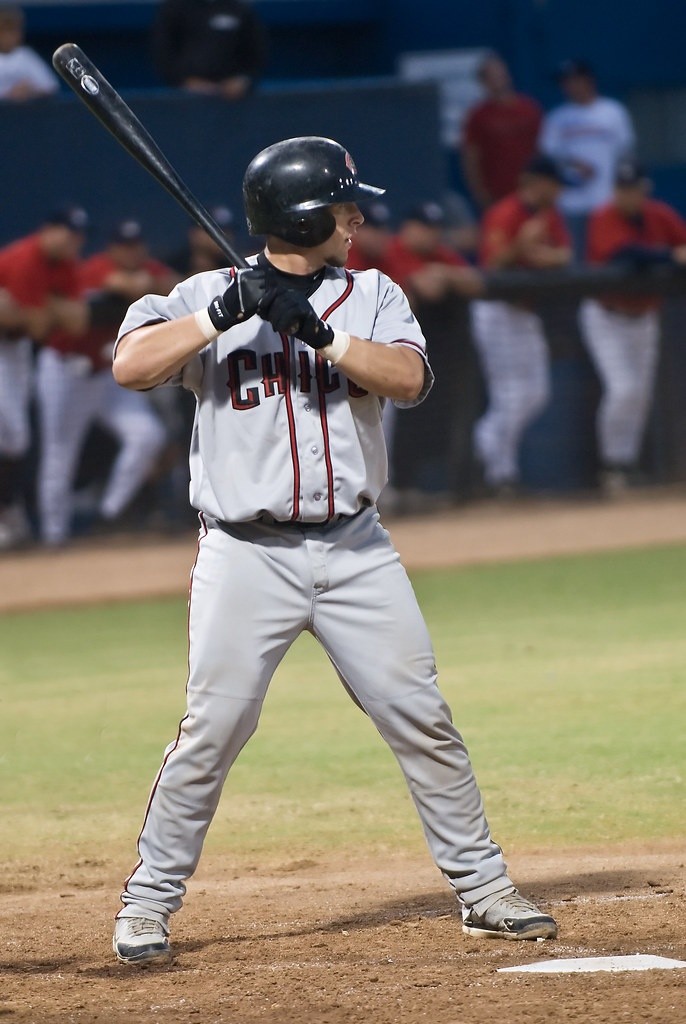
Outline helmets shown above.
[243,137,387,248]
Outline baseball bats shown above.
[51,41,305,339]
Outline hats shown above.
[526,154,576,190]
[205,205,237,230]
[117,218,148,245]
[51,204,91,233]
[614,161,648,186]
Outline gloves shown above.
[208,264,277,332]
[257,290,335,351]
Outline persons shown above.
[0,192,478,558]
[534,62,637,220]
[456,51,541,216]
[112,139,560,964]
[467,153,572,496]
[157,0,258,100]
[577,154,686,491]
[0,0,62,104]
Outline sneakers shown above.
[461,893,556,941]
[114,916,172,965]
